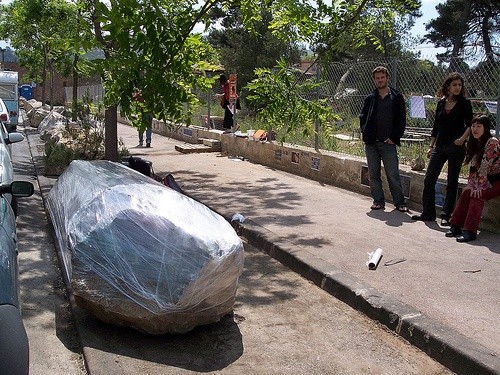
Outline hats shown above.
[219,75,228,84]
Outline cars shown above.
[0,65,34,375]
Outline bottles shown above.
[247,130,255,141]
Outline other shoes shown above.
[441,218,450,226]
[446,227,462,237]
[412,214,435,222]
[233,124,240,133]
[223,129,232,133]
[456,232,476,242]
[146,143,150,147]
[137,144,143,146]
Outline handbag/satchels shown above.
[252,130,277,141]
[220,96,227,109]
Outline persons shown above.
[137,108,153,148]
[214,74,240,134]
[411,73,474,224]
[359,65,408,211]
[445,114,500,242]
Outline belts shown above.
[373,137,386,142]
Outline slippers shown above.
[396,206,409,212]
[371,204,384,209]
